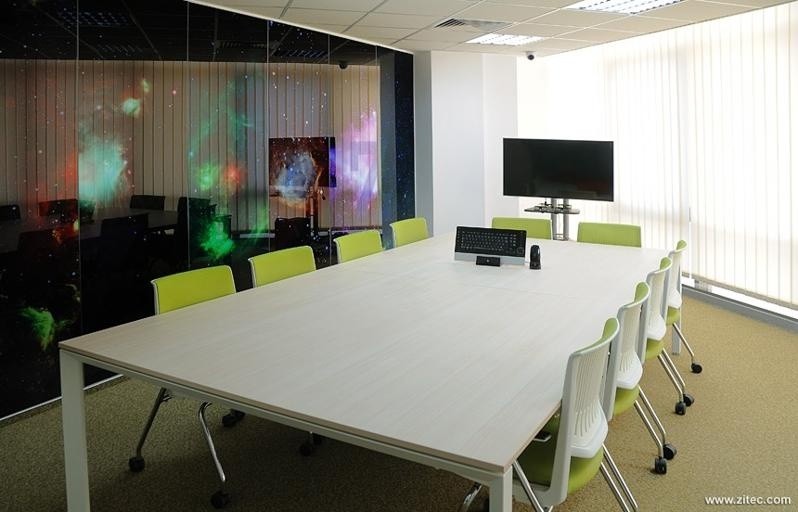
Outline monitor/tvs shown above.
[502,137,614,202]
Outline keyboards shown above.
[455,225,527,257]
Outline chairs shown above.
[637,258,686,459]
[493,217,553,240]
[663,239,702,405]
[0,196,316,419]
[389,218,430,247]
[599,283,667,511]
[578,222,642,247]
[462,315,630,512]
[332,230,383,263]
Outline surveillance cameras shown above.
[526,52,535,62]
[338,57,350,70]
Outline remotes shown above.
[476,254,502,266]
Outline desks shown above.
[58,233,663,512]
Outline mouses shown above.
[529,244,542,269]
